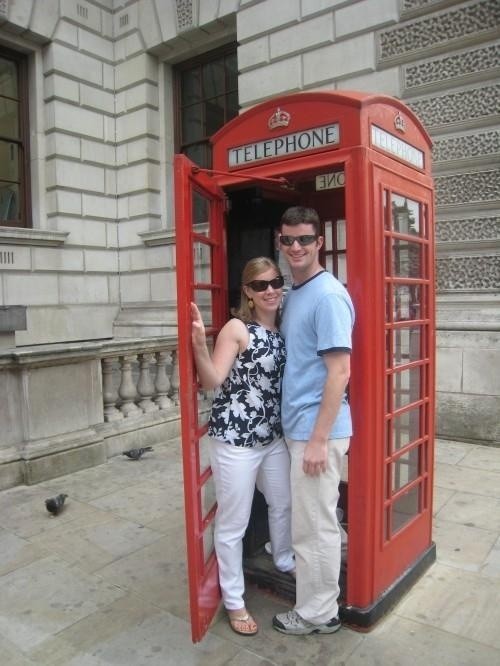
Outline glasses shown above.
[245,275,285,293]
[278,233,319,247]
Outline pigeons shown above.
[43,493,69,516]
[120,446,153,462]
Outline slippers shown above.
[227,608,258,636]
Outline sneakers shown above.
[270,608,342,636]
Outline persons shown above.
[274,205,356,635]
[188,256,297,638]
